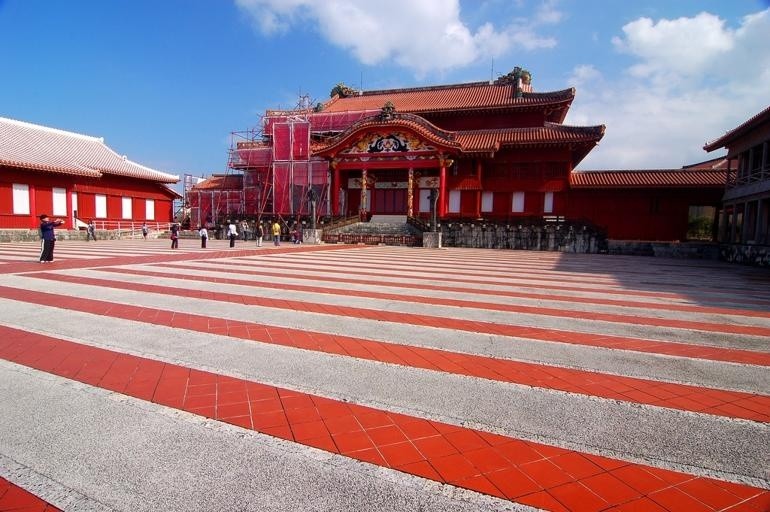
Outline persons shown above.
[142,222,149,239]
[271,219,283,245]
[171,224,180,249]
[255,223,264,246]
[228,220,239,248]
[85,219,97,241]
[289,228,302,244]
[241,219,250,241]
[199,224,209,249]
[37,214,65,264]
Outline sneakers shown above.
[41,260,45,263]
[46,260,54,263]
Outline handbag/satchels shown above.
[171,234,176,240]
[256,232,262,237]
[231,234,236,238]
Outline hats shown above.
[40,215,48,219]
